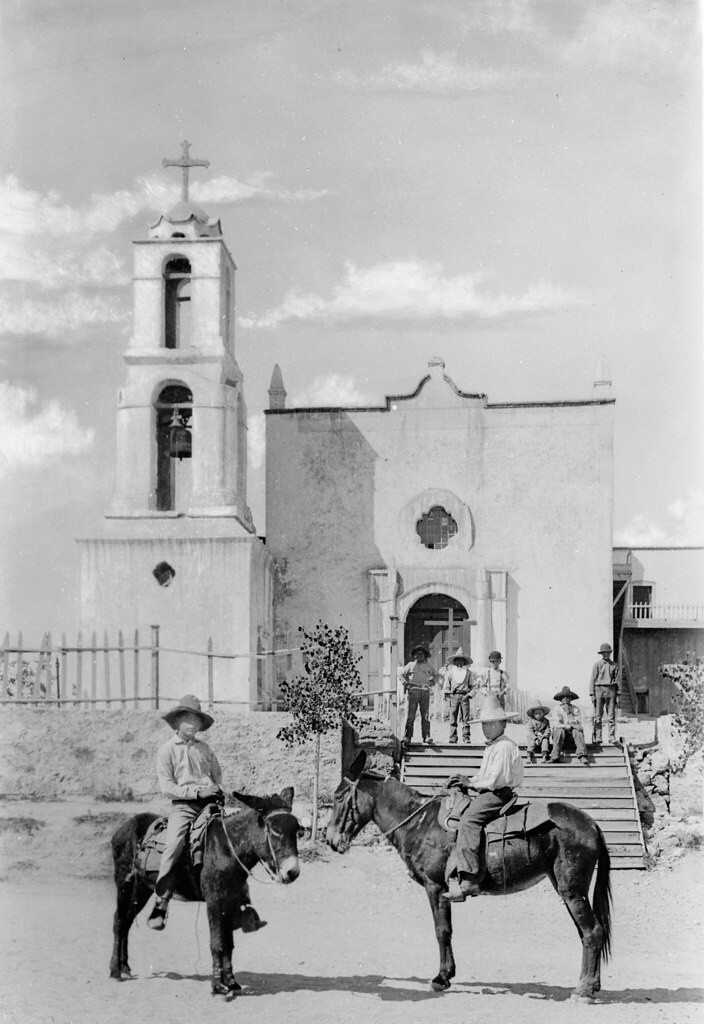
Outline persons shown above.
[526,699,551,763]
[399,645,436,743]
[442,696,523,898]
[479,651,510,710]
[147,694,267,933]
[546,687,590,764]
[443,647,476,744]
[589,643,622,744]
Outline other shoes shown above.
[150,897,169,929]
[546,758,562,764]
[609,739,623,745]
[541,756,547,764]
[526,757,535,764]
[443,879,485,898]
[580,757,591,765]
[255,918,267,930]
[594,738,603,744]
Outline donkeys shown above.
[108,786,300,1001]
[325,749,610,1005]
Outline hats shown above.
[553,686,580,701]
[526,698,553,717]
[489,651,504,660]
[447,647,474,663]
[597,643,617,654]
[161,694,214,733]
[468,696,520,724]
[411,644,434,658]
